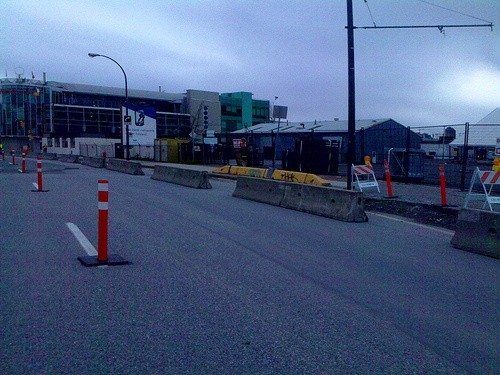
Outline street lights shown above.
[87,52,131,160]
